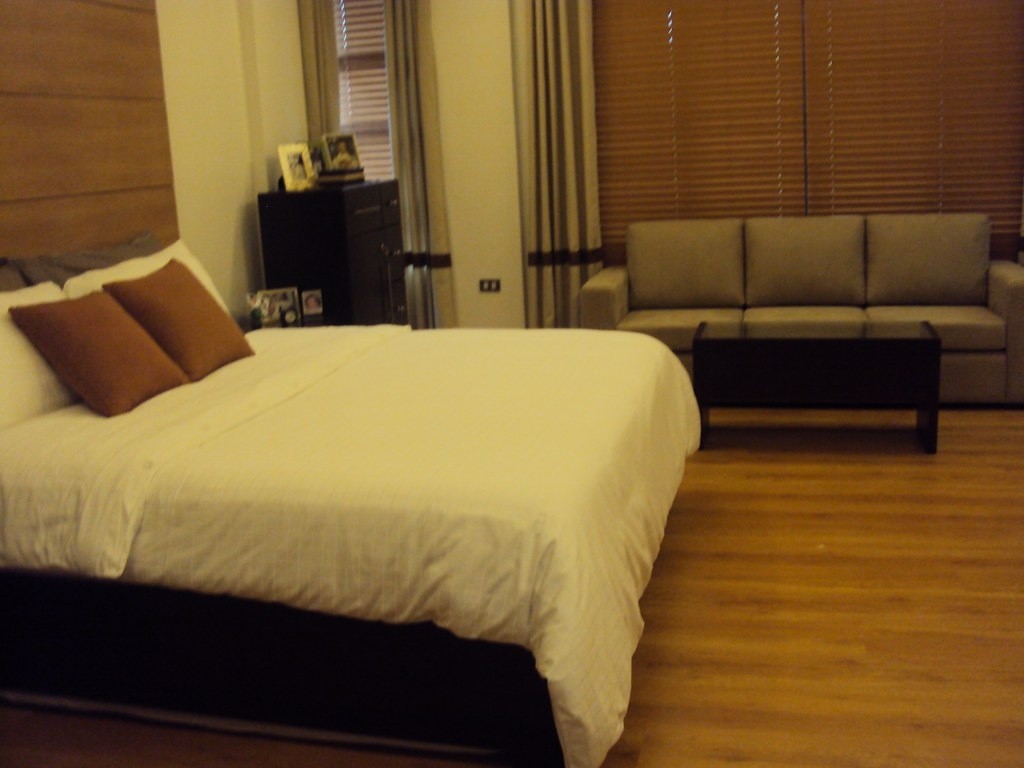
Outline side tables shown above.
[694,317,942,455]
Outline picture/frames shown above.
[256,287,301,326]
[321,132,365,185]
[300,289,323,315]
[278,142,318,192]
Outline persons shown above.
[308,145,326,172]
[331,138,359,170]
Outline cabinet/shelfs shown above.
[257,179,405,326]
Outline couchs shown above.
[577,210,1024,405]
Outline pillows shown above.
[9,291,195,419]
[103,257,255,379]
[63,240,231,315]
[0,279,82,429]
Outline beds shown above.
[0,326,705,766]
